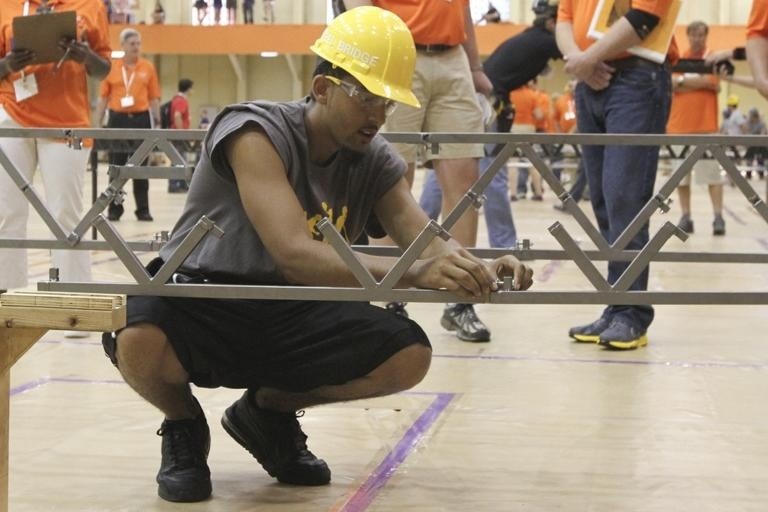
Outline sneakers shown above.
[676,219,693,233]
[156,394,213,502]
[220,392,330,485]
[568,318,608,342]
[598,320,647,350]
[713,220,724,234]
[441,302,488,342]
[139,214,150,220]
[109,213,117,219]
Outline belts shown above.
[417,44,448,52]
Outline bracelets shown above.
[4,58,16,73]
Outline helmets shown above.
[309,5,422,108]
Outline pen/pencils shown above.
[57,38,76,69]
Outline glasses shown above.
[324,75,398,116]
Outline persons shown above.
[552,0,671,350]
[197,109,210,128]
[152,0,166,23]
[109,6,534,494]
[0,0,112,337]
[476,2,503,24]
[193,0,280,24]
[420,0,592,248]
[161,77,198,193]
[666,0,768,235]
[96,28,160,220]
[344,0,495,342]
